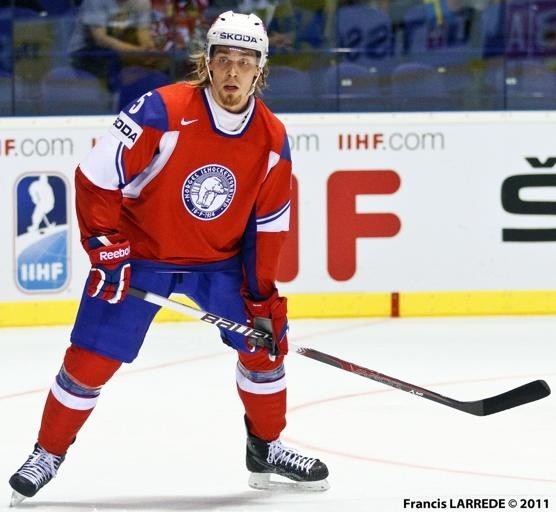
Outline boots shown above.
[9,435,76,497]
[244,414,329,482]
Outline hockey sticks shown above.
[127,285,551,416]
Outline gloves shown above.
[243,287,288,362]
[82,233,132,305]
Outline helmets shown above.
[204,10,270,72]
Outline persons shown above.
[10,11,330,497]
[0,0,556,112]
